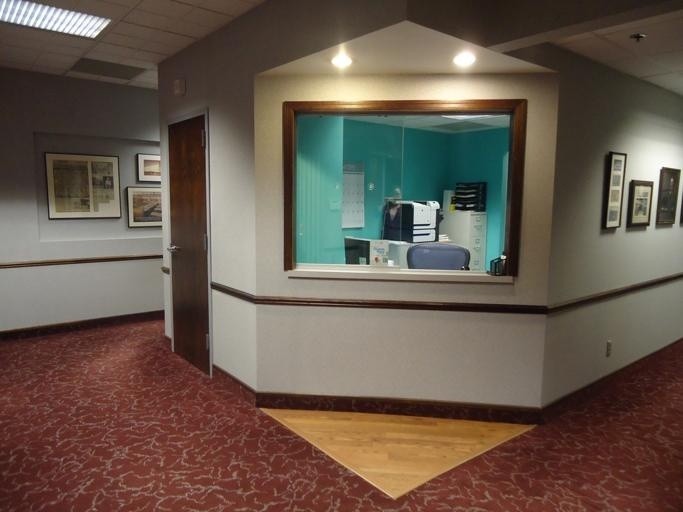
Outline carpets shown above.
[259,407,538,500]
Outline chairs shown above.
[407,244,470,270]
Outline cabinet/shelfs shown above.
[439,212,487,271]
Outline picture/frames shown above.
[602,151,683,229]
[44,152,162,228]
